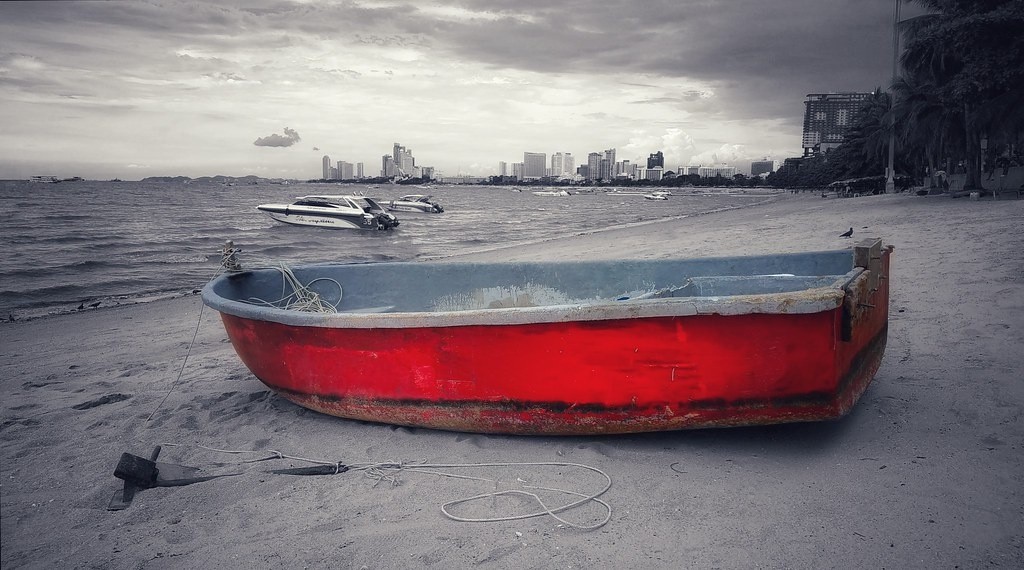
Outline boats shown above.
[653,189,673,196]
[198,238,896,437]
[376,193,444,214]
[645,193,669,200]
[254,191,402,231]
[531,189,572,197]
